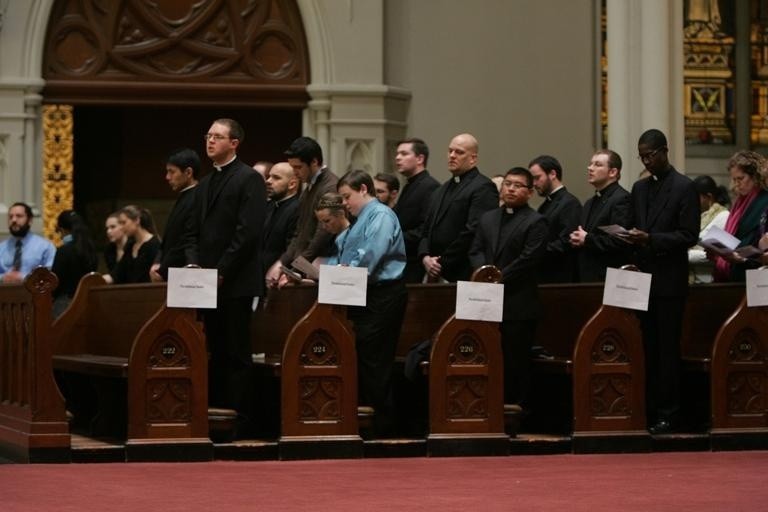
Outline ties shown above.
[13,240,23,271]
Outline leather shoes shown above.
[647,418,669,435]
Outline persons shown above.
[391,128,768,438]
[0,201,57,284]
[50,116,407,438]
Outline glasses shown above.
[503,181,527,190]
[638,146,661,160]
[203,135,230,141]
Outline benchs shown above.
[251,263,364,444]
[51,272,213,447]
[680,252,768,436]
[531,264,651,438]
[394,264,510,441]
